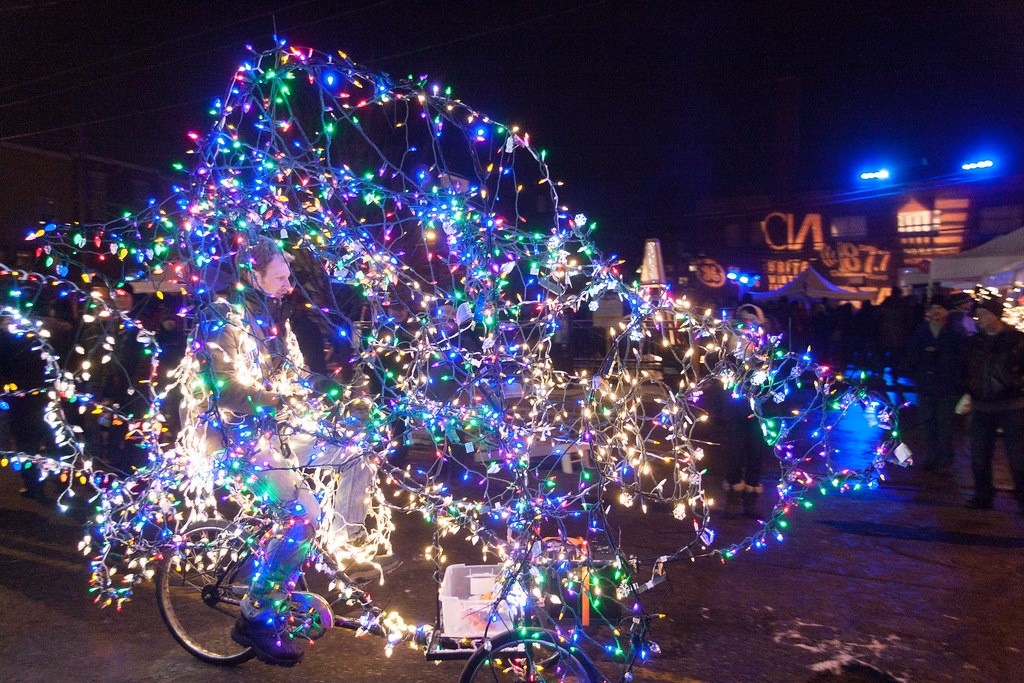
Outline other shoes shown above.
[970,490,993,509]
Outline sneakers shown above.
[343,553,404,585]
[232,611,304,668]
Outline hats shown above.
[930,295,949,309]
[976,300,1004,318]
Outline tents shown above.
[896,227,1024,306]
[748,264,880,353]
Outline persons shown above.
[902,292,968,474]
[361,289,421,469]
[712,301,774,523]
[175,231,407,669]
[955,294,1024,513]
[0,306,82,509]
[83,280,166,471]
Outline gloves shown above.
[307,380,343,420]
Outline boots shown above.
[741,482,766,519]
[723,478,744,519]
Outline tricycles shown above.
[153,379,624,683]
